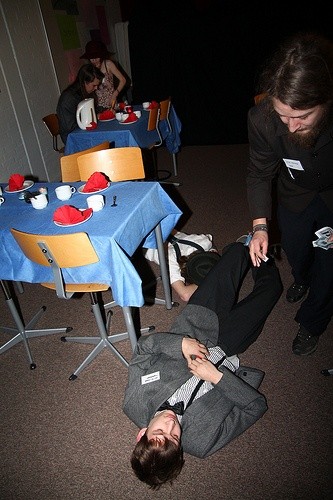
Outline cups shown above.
[116,113,124,121]
[133,111,142,118]
[55,185,77,201]
[0,196,4,206]
[0,187,3,196]
[30,194,48,210]
[121,114,130,121]
[38,186,48,195]
[143,102,152,109]
[87,194,105,211]
[124,106,132,112]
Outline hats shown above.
[186,251,221,287]
[79,39,116,59]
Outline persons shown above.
[80,40,126,111]
[246,37,333,354]
[123,241,283,489]
[56,64,105,147]
[140,228,222,302]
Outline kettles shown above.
[76,98,97,130]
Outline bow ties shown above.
[158,400,185,416]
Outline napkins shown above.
[53,205,92,225]
[122,112,137,123]
[119,103,127,110]
[84,171,108,192]
[98,108,116,120]
[147,101,159,109]
[8,174,25,190]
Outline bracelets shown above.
[252,223,268,233]
[116,88,120,94]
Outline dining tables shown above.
[0,181,183,355]
[63,103,191,153]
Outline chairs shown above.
[59,141,179,312]
[42,114,66,153]
[142,96,178,180]
[0,275,73,368]
[8,227,156,380]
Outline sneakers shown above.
[293,324,320,356]
[287,282,308,304]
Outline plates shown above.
[97,115,116,121]
[54,208,92,228]
[77,182,111,194]
[119,119,138,124]
[3,181,34,194]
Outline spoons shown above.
[111,195,117,207]
